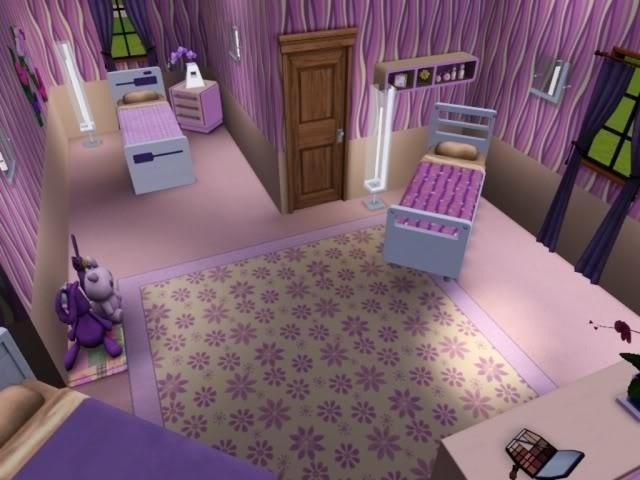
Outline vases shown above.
[185,62,206,88]
[627,372,640,412]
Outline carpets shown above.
[115,212,557,480]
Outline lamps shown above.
[360,81,397,211]
[52,40,103,149]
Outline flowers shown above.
[170,46,197,65]
[588,319,640,372]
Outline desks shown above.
[428,353,640,480]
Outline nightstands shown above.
[168,79,224,134]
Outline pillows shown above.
[123,89,159,105]
[434,141,479,161]
[0,383,45,450]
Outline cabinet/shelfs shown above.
[373,51,480,95]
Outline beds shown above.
[383,101,498,282]
[106,66,197,196]
[1,325,275,480]
